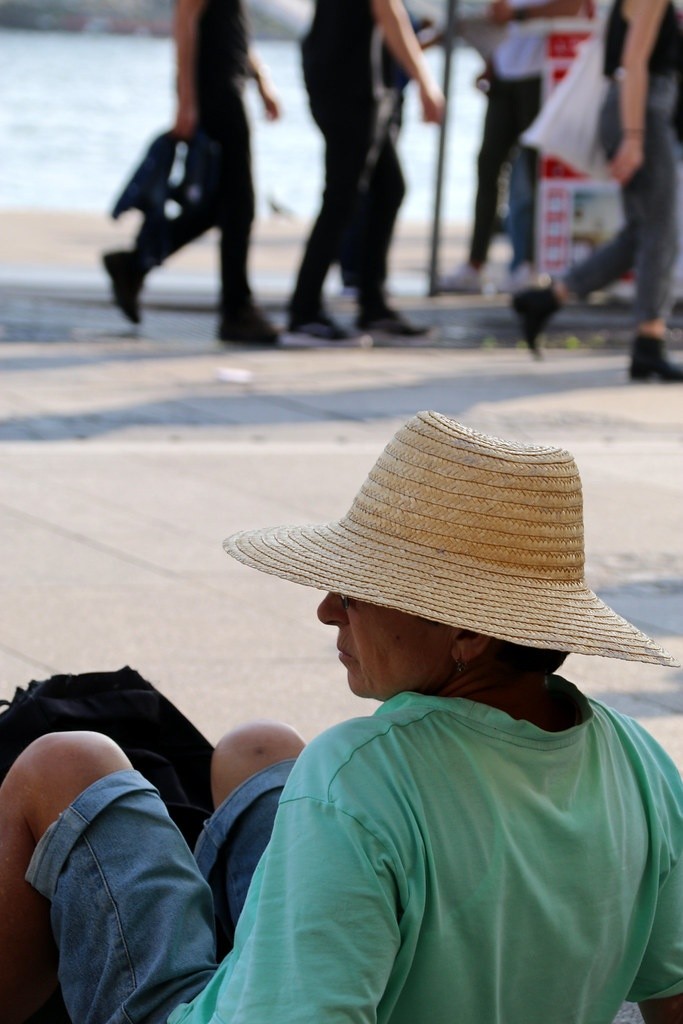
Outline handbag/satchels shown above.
[520,0,612,185]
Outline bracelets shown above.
[620,129,644,140]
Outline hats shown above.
[222,411,681,668]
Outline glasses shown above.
[342,595,349,608]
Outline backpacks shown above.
[0,667,225,1024]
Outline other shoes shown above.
[357,303,429,335]
[500,270,531,293]
[629,337,683,381]
[104,248,150,323]
[219,301,278,343]
[510,284,560,353]
[438,267,484,293]
[289,313,344,339]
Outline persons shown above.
[0,410,682,1024]
[103,1,284,351]
[276,1,683,385]
